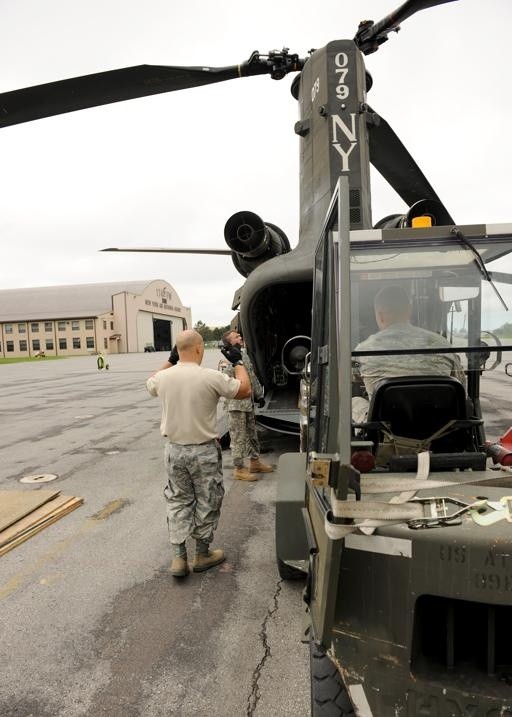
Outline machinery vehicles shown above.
[34,349,46,357]
[278,174,512,717]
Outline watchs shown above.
[232,359,245,367]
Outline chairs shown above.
[353,376,484,452]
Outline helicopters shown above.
[0,0,512,441]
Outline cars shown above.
[204,340,224,348]
[144,345,155,352]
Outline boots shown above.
[193,543,224,572]
[233,468,256,480]
[170,544,190,577]
[248,459,272,473]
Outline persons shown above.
[217,330,273,482]
[347,286,469,436]
[145,329,252,580]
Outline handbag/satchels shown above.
[376,435,431,467]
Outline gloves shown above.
[221,345,242,363]
[168,344,179,364]
[257,398,266,409]
